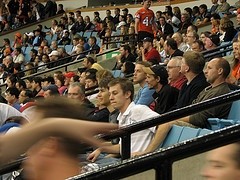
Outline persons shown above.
[86,77,173,162]
[0,0,240,180]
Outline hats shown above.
[64,72,77,78]
[27,32,34,37]
[141,37,152,42]
[4,48,11,54]
[14,33,22,39]
[142,65,168,79]
[205,34,220,46]
[117,21,126,28]
[222,11,232,15]
[48,50,59,57]
[122,8,129,13]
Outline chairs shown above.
[0,9,240,180]
